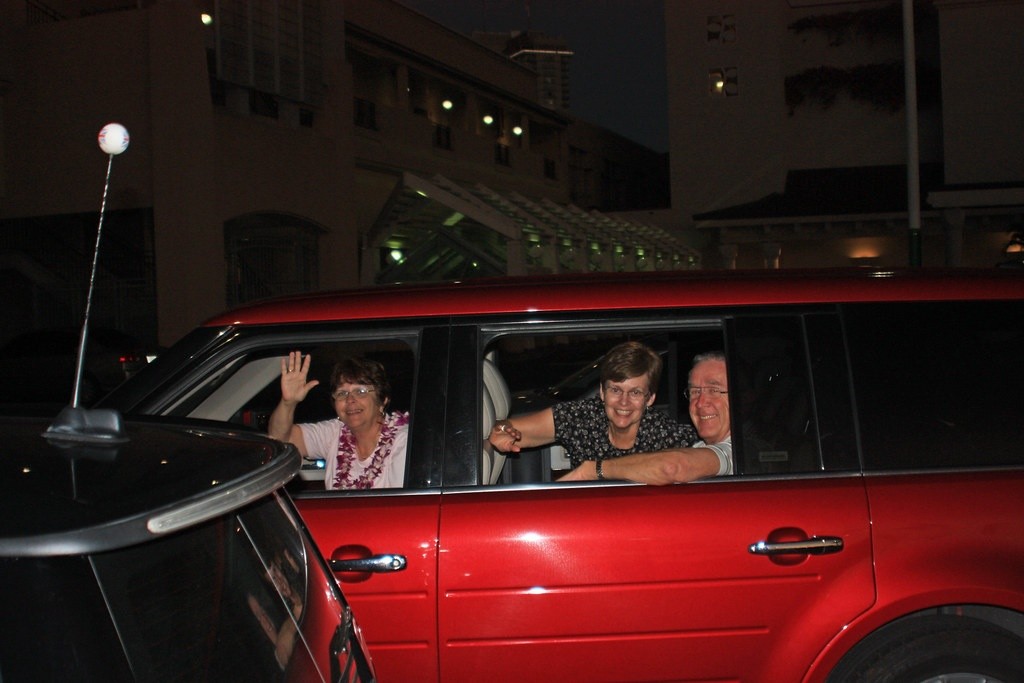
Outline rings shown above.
[501,425,505,432]
[288,369,295,373]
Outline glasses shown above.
[332,387,375,401]
[604,387,649,401]
[684,387,728,400]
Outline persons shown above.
[269,350,410,490]
[489,341,733,484]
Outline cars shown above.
[0,403,380,683]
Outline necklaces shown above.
[333,412,409,491]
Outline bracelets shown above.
[595,455,607,480]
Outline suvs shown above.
[0,262,1023,683]
